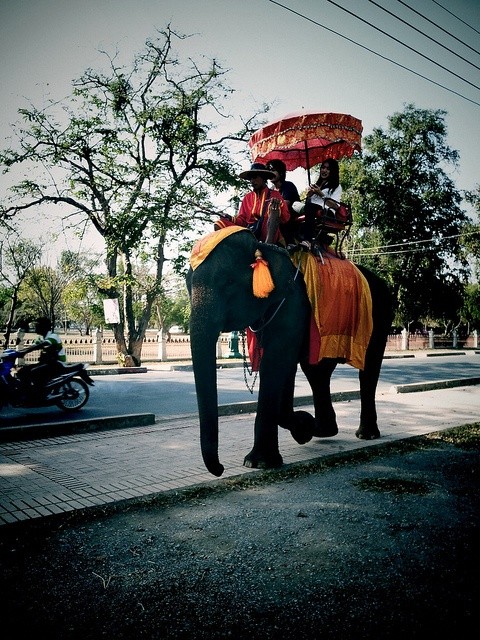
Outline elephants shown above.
[186,226,395,476]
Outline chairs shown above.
[296,193,354,261]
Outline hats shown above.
[240,165,274,179]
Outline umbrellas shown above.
[248,108,364,199]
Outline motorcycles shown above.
[0,336,96,412]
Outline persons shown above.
[17,318,67,390]
[262,159,303,215]
[306,158,344,226]
[214,163,291,244]
